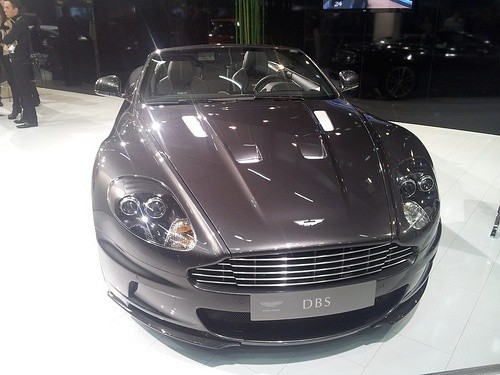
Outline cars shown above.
[327,28,498,102]
[17,24,88,40]
[91,43,441,353]
[207,18,246,43]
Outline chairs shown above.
[158,61,208,94]
[231,51,276,90]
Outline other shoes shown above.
[7,105,23,119]
[13,118,26,124]
[16,120,37,128]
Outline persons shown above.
[0,0,41,128]
